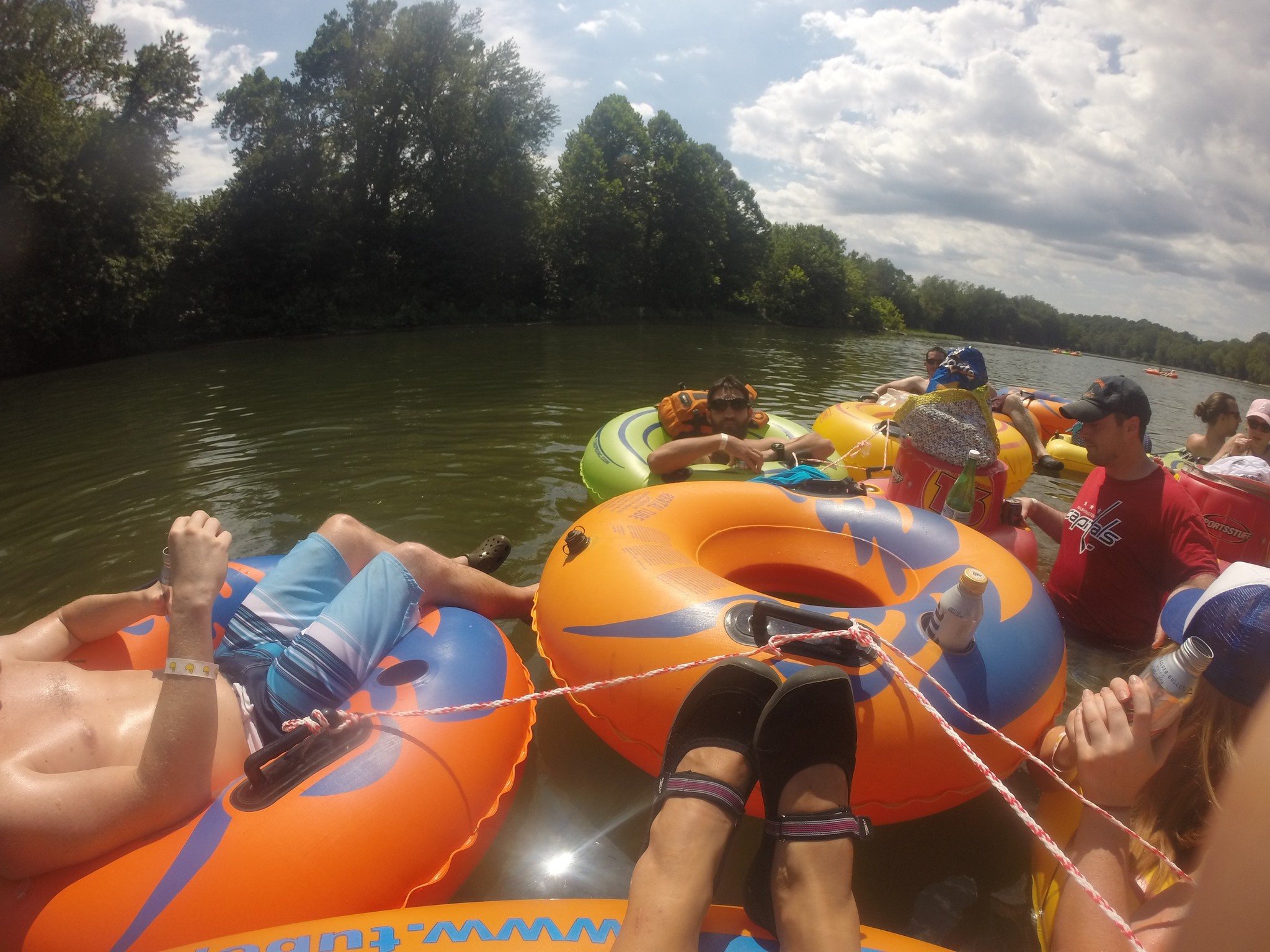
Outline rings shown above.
[1241,441,1243,443]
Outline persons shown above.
[609,345,1270,952]
[0,510,540,889]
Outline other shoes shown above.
[647,657,782,888]
[1036,454,1064,471]
[743,665,872,939]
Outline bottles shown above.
[925,567,989,653]
[159,546,173,619]
[1121,635,1214,742]
[941,449,980,526]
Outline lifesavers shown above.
[1031,688,1216,952]
[1046,430,1104,475]
[158,892,963,952]
[1159,446,1196,476]
[0,557,544,950]
[997,384,1083,436]
[1146,368,1178,378]
[577,397,846,504]
[813,402,1032,499]
[512,476,1067,825]
[1051,348,1084,357]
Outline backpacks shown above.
[657,382,770,439]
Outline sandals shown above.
[459,535,512,575]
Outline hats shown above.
[1161,561,1270,705]
[1246,398,1270,425]
[1059,375,1152,425]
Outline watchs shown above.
[771,443,785,462]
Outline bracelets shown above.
[1229,452,1232,457]
[1032,498,1038,517]
[162,656,222,681]
[718,433,729,452]
[1052,732,1078,774]
[870,391,880,398]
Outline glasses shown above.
[927,359,944,365]
[712,398,749,410]
[1247,418,1270,434]
